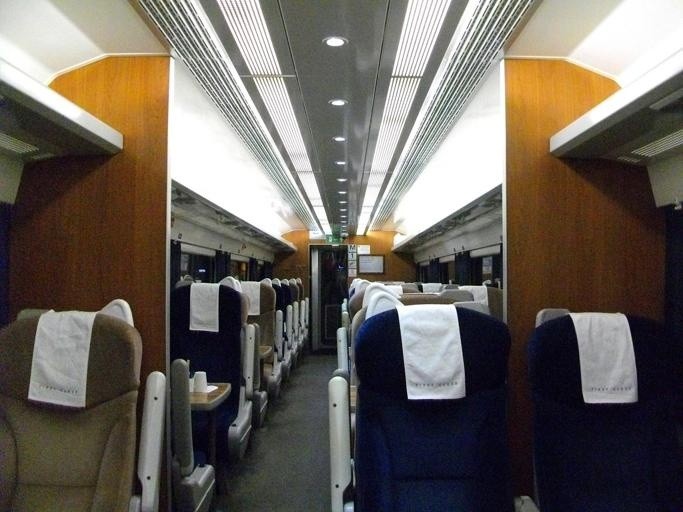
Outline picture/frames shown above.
[357,254,384,275]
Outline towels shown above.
[27,309,98,409]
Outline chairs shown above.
[531,307,683,511]
[327,275,538,511]
[0,298,166,512]
[172,274,311,512]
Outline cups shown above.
[193,371,207,393]
